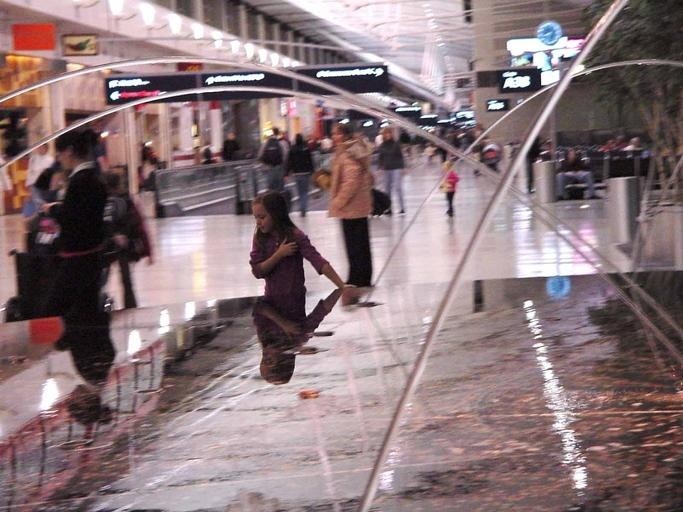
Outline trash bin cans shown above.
[532,161,558,203]
[607,176,640,243]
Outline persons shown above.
[102,169,153,311]
[527,130,648,195]
[30,126,112,314]
[407,117,506,175]
[371,126,410,214]
[245,187,359,296]
[441,162,459,218]
[251,296,347,387]
[262,125,315,216]
[138,127,247,170]
[3,103,42,159]
[51,309,120,430]
[24,137,52,189]
[323,117,382,290]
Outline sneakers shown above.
[398,208,406,214]
[385,209,391,215]
[300,209,306,217]
[446,208,453,217]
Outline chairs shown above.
[498,128,678,203]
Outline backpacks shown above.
[264,138,282,166]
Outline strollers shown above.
[474,141,502,177]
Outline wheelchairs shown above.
[3,245,114,325]
